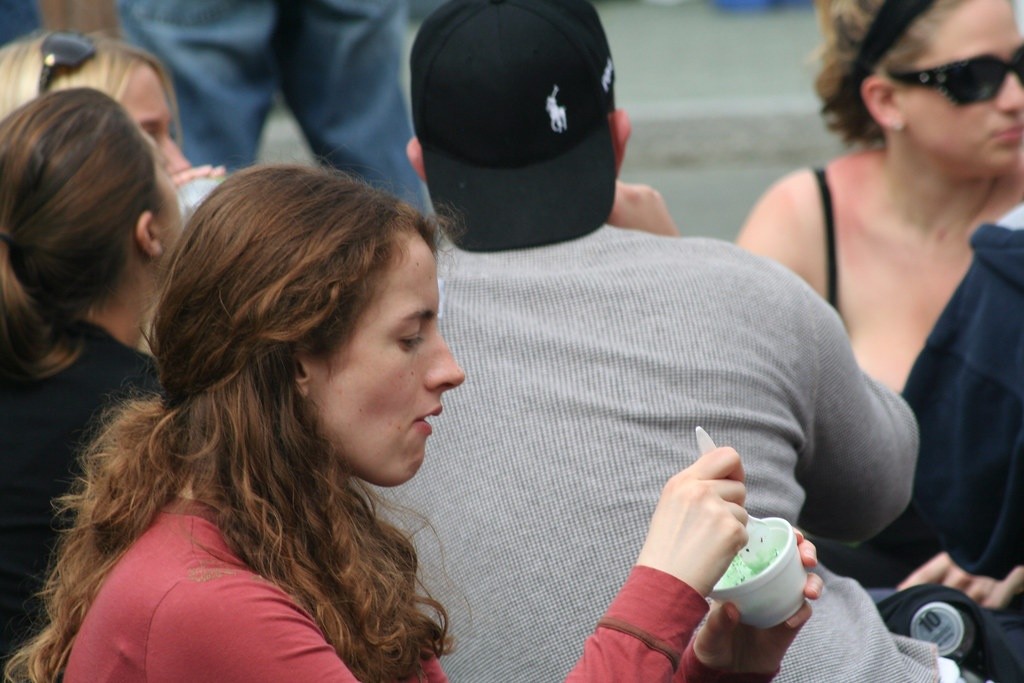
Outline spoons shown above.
[695,425,776,572]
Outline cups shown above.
[708,517,808,629]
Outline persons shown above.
[0,83,187,673]
[732,0,1024,683]
[0,161,823,683]
[2,32,228,190]
[112,0,434,221]
[349,0,994,683]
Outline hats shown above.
[410,0,616,252]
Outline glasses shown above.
[884,41,1024,104]
[35,31,96,93]
